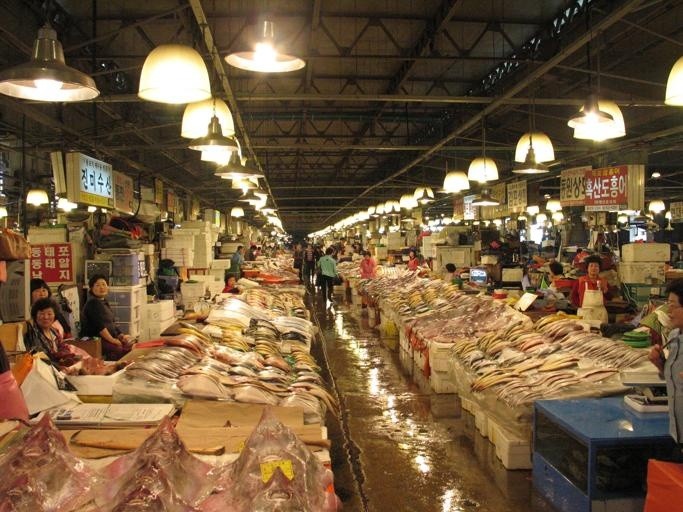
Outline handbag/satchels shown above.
[332,275,343,286]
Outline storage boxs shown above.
[63,223,228,360]
[395,326,533,471]
[618,243,671,285]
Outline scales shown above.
[620,372,670,413]
[183,300,209,318]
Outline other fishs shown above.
[0,407,99,512]
[90,407,224,512]
[204,407,344,511]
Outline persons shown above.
[572,255,611,326]
[28,278,72,339]
[79,274,133,362]
[222,237,421,305]
[548,260,564,289]
[444,262,457,282]
[662,276,683,462]
[573,249,583,268]
[23,298,77,372]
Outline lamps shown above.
[136,14,305,219]
[0,2,99,107]
[24,188,77,212]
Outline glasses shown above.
[664,300,680,309]
